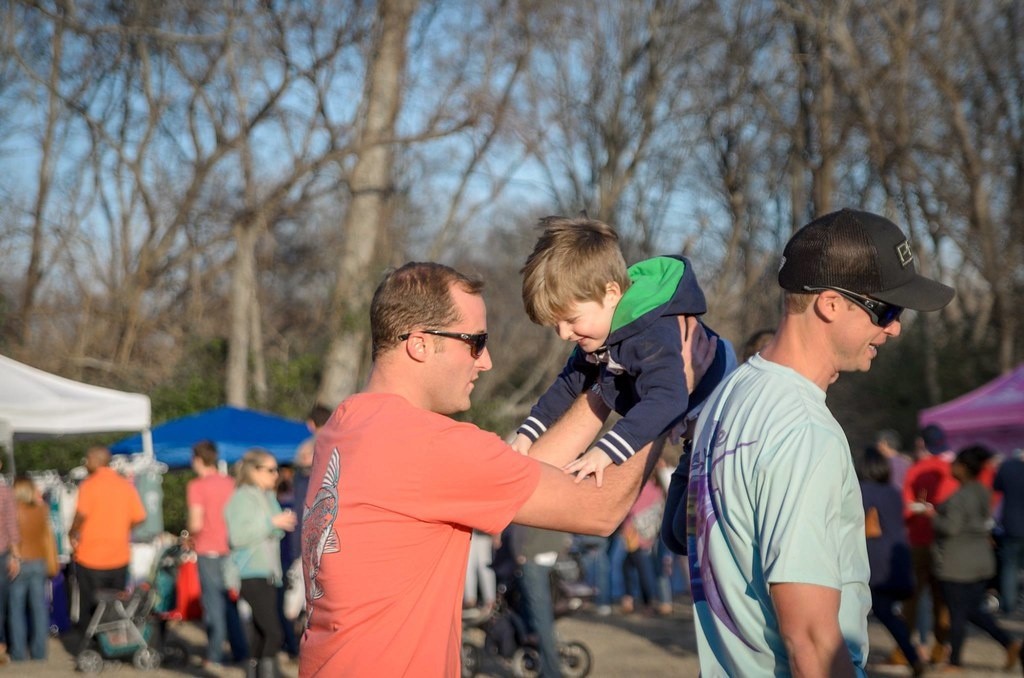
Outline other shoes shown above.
[931,642,950,665]
[941,665,967,672]
[1004,639,1023,672]
[889,646,924,665]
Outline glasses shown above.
[255,463,280,473]
[398,329,488,359]
[803,285,904,328]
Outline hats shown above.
[778,207,956,312]
[918,424,949,455]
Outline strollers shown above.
[457,540,592,677]
[72,537,184,675]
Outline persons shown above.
[889,422,962,664]
[288,403,331,560]
[510,525,568,678]
[69,445,147,626]
[185,437,251,671]
[507,221,742,555]
[300,264,716,677]
[851,449,930,677]
[463,532,495,607]
[0,465,87,662]
[875,429,912,492]
[222,449,295,677]
[689,208,957,677]
[988,448,1020,617]
[921,444,1022,671]
[564,452,694,616]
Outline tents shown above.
[916,364,1024,443]
[109,403,312,467]
[0,356,155,469]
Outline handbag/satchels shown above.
[221,554,242,600]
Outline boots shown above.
[246,658,285,678]
